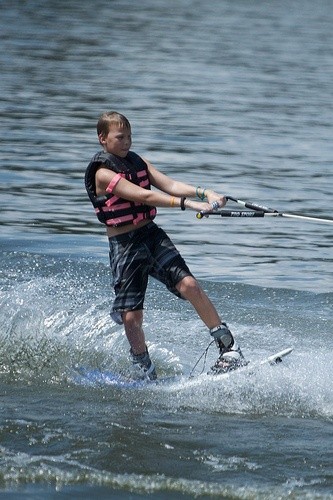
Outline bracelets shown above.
[196,186,207,200]
[170,195,174,208]
[180,196,187,211]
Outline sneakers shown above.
[207,323,248,376]
[129,345,157,382]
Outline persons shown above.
[84,111,247,383]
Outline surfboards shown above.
[65,345,293,395]
